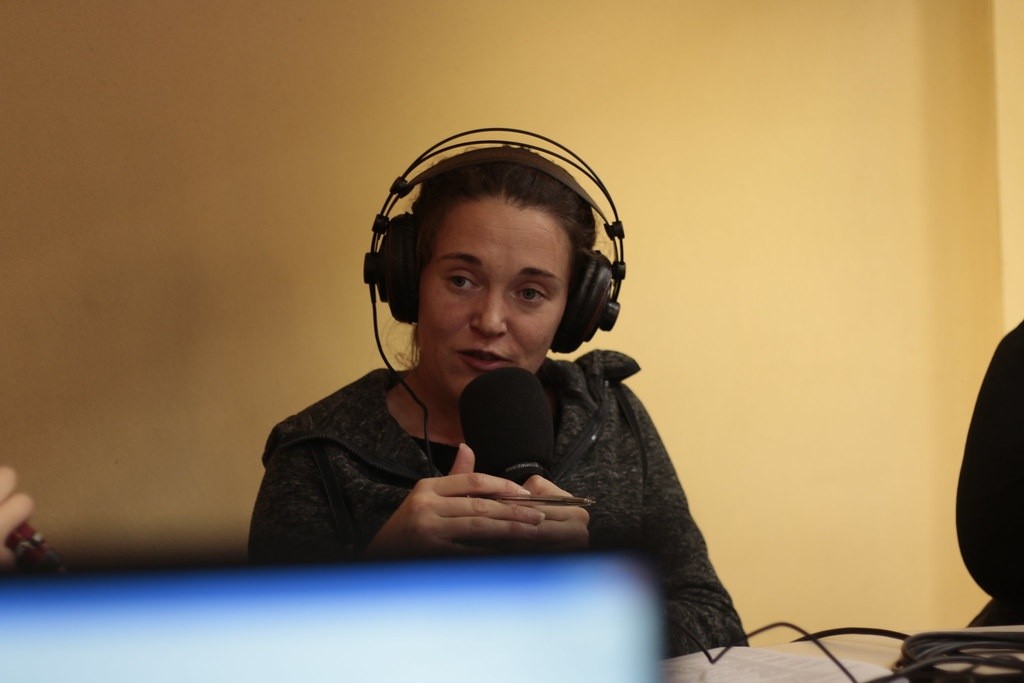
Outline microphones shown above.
[459,368,551,487]
[4,520,64,563]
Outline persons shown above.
[1,467,33,574]
[955,319,1023,630]
[247,145,749,660]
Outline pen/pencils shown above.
[467,492,599,509]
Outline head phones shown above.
[364,128,625,354]
